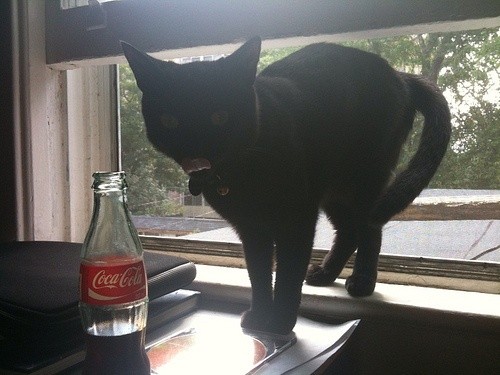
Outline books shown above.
[0,286,201,375]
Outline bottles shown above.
[77,170,153,375]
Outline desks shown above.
[63,305,357,374]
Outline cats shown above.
[121,34,451,335]
